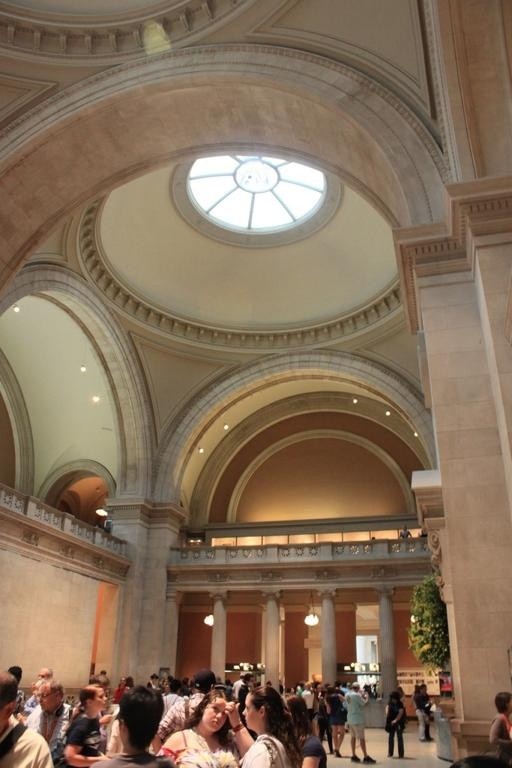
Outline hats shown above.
[390,691,400,698]
[195,670,215,690]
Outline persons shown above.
[419,527,427,537]
[449,753,510,767]
[1,666,375,768]
[490,691,512,766]
[384,683,437,758]
[399,525,412,538]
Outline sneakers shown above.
[351,755,360,761]
[364,756,376,763]
